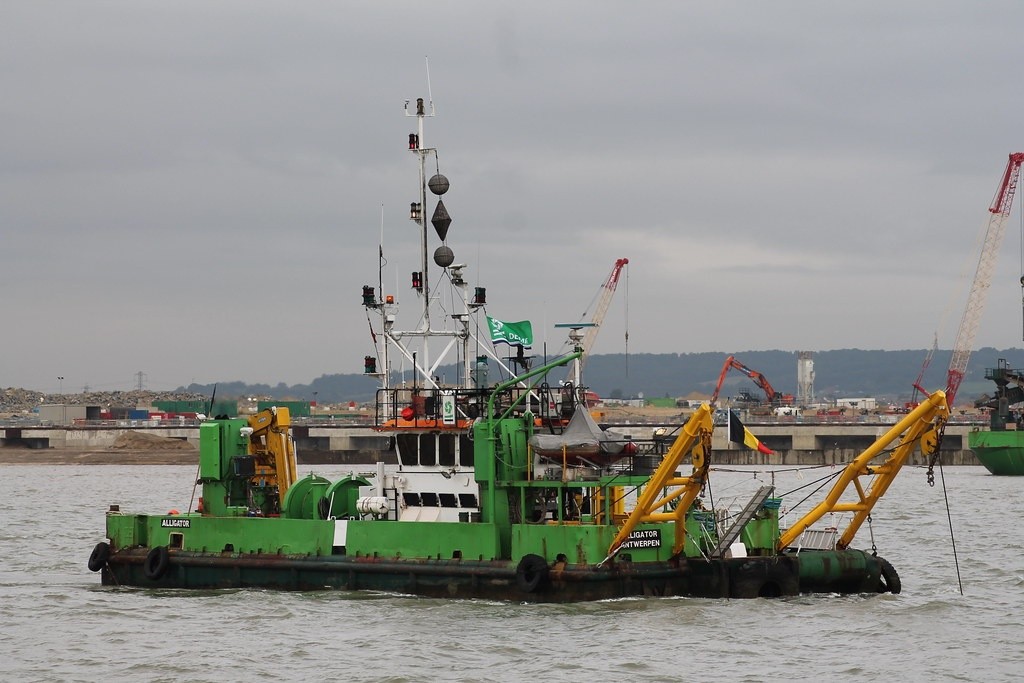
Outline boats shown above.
[965,274,1024,477]
[87,50,956,597]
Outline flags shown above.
[728,408,775,456]
[486,316,533,350]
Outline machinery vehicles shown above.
[709,354,796,415]
[552,256,631,426]
[888,150,1024,419]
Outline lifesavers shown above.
[517,554,549,593]
[144,546,169,579]
[873,556,901,595]
[88,542,109,572]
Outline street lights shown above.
[57,376,64,404]
